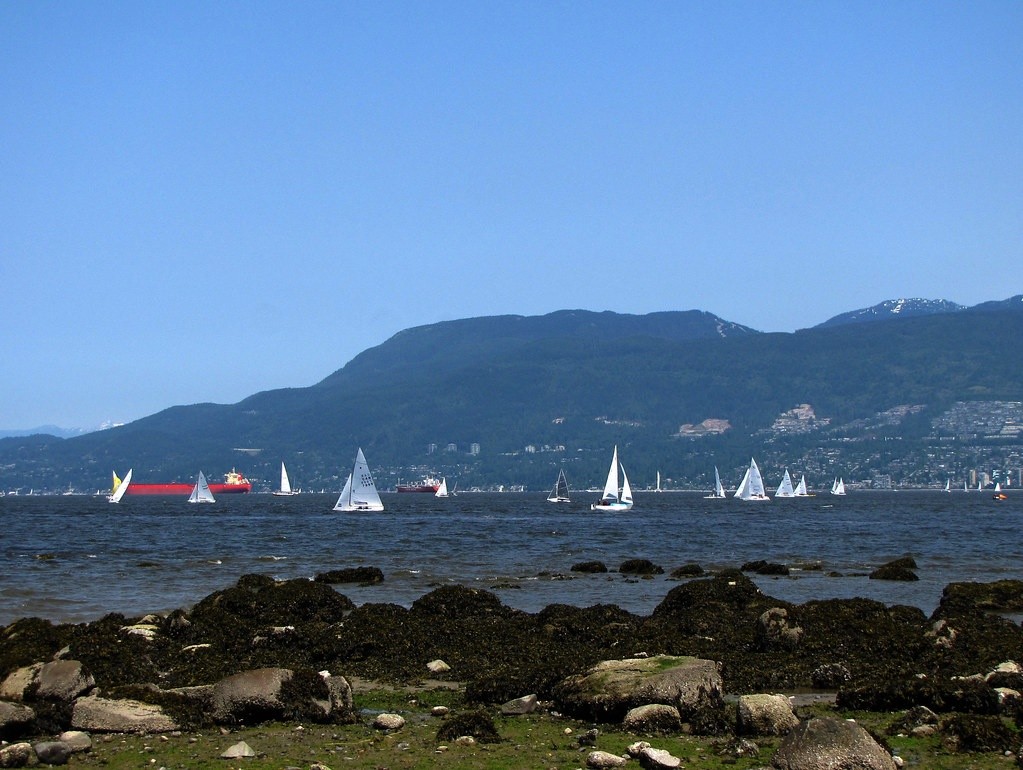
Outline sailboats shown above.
[775,469,796,497]
[435,476,449,498]
[703,464,727,499]
[546,468,571,503]
[940,477,1001,493]
[733,458,770,500]
[273,460,294,496]
[793,474,816,498]
[108,469,133,505]
[589,445,634,511]
[829,476,848,496]
[333,448,386,513]
[186,470,217,504]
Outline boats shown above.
[125,466,252,497]
[395,475,441,492]
